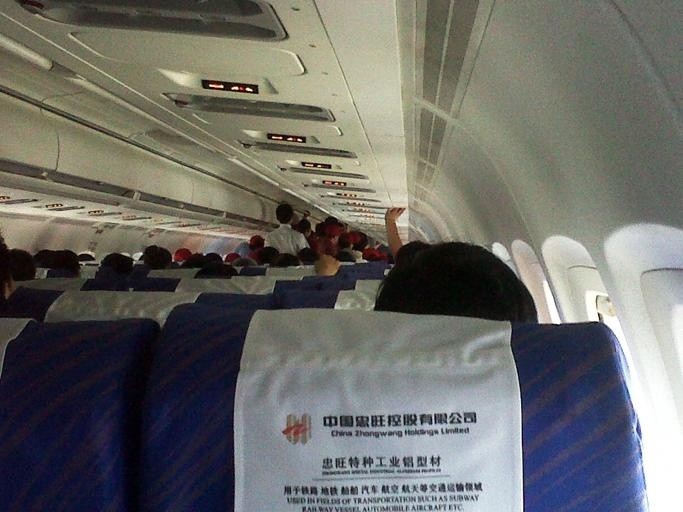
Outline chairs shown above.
[1,263,645,511]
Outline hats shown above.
[375,240,538,325]
[175,215,390,267]
